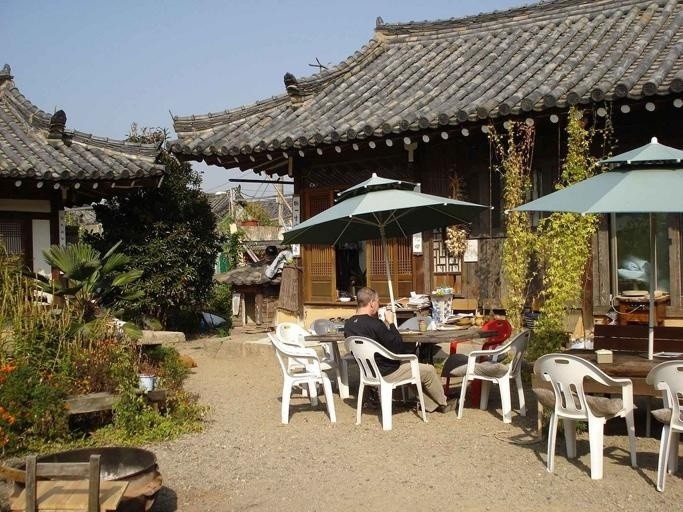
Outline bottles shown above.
[326,316,344,336]
[335,292,351,302]
[418,320,427,331]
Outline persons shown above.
[343,287,459,413]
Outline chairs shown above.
[646,357,683,494]
[27,455,103,512]
[266,319,529,432]
[530,351,639,481]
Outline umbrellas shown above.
[509,136,683,437]
[279,172,493,404]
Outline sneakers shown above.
[440,398,458,412]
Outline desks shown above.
[556,352,683,397]
[616,296,669,325]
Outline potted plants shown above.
[137,361,156,392]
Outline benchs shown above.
[593,326,683,363]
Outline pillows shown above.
[650,402,683,431]
[532,373,624,430]
